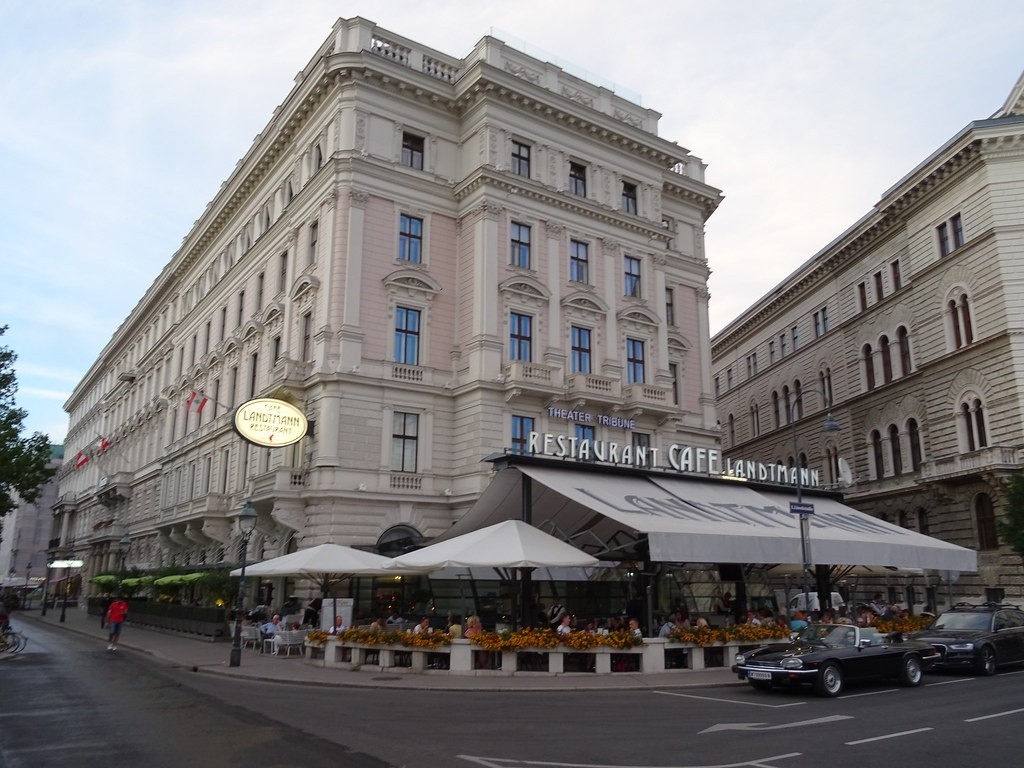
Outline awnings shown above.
[49,573,80,584]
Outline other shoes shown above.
[107,643,113,650]
[113,646,117,651]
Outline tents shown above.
[418,449,978,610]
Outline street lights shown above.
[789,388,841,627]
[59,549,77,622]
[228,499,258,668]
[41,559,54,617]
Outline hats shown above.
[669,615,676,622]
[792,612,806,621]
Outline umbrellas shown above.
[230,541,433,597]
[383,518,600,632]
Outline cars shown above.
[732,623,943,697]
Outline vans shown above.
[784,592,847,612]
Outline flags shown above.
[75,451,90,468]
[186,391,207,413]
[98,436,112,450]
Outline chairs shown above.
[230,622,317,656]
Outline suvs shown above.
[911,601,1024,676]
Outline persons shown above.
[106,594,128,651]
[234,590,886,653]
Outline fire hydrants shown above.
[22,562,32,610]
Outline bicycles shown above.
[0,626,29,654]
